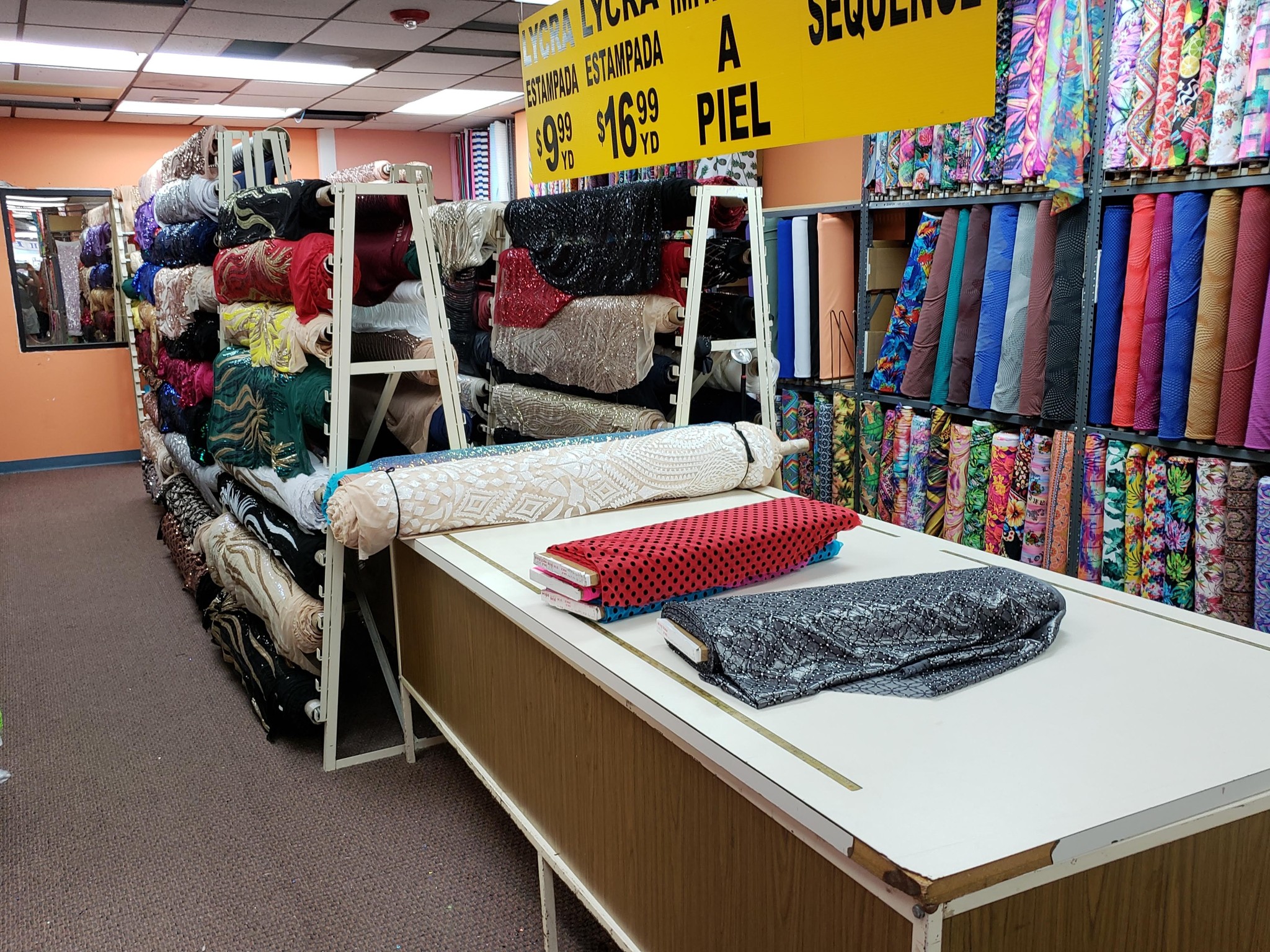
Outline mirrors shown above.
[0,187,130,354]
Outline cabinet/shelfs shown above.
[128,0,1270,774]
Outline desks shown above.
[387,476,1270,952]
